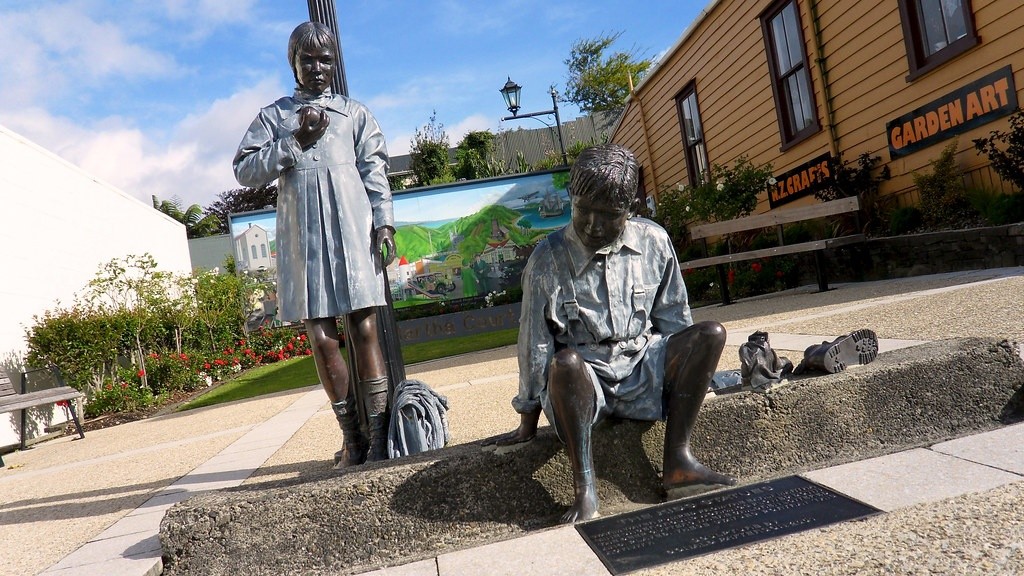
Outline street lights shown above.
[497,75,570,167]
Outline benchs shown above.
[679,196,865,310]
[0,364,86,468]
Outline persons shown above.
[481,143,740,522]
[232,21,397,466]
